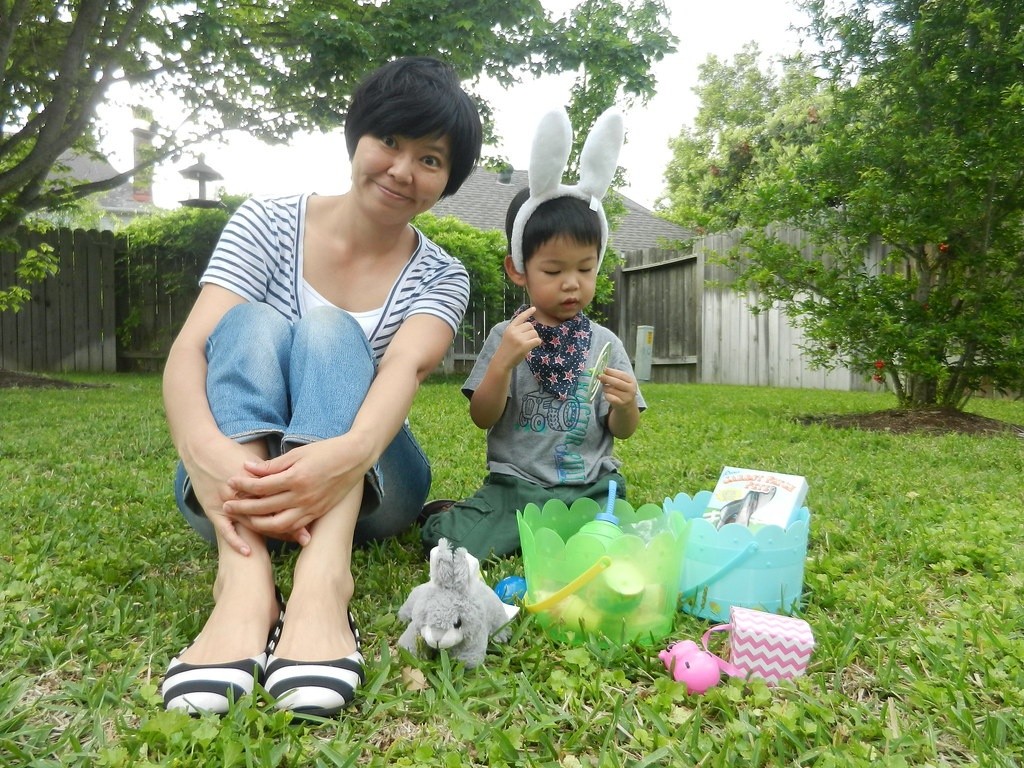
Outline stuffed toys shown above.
[395,539,513,670]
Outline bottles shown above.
[578,514,623,549]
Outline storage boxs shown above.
[701,605,814,685]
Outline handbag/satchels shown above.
[700,605,813,686]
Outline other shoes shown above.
[265,600,364,725]
[161,585,285,714]
[415,498,457,528]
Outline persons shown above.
[417,108,647,572]
[161,55,470,722]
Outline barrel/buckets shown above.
[662,490,810,623]
[516,498,694,644]
